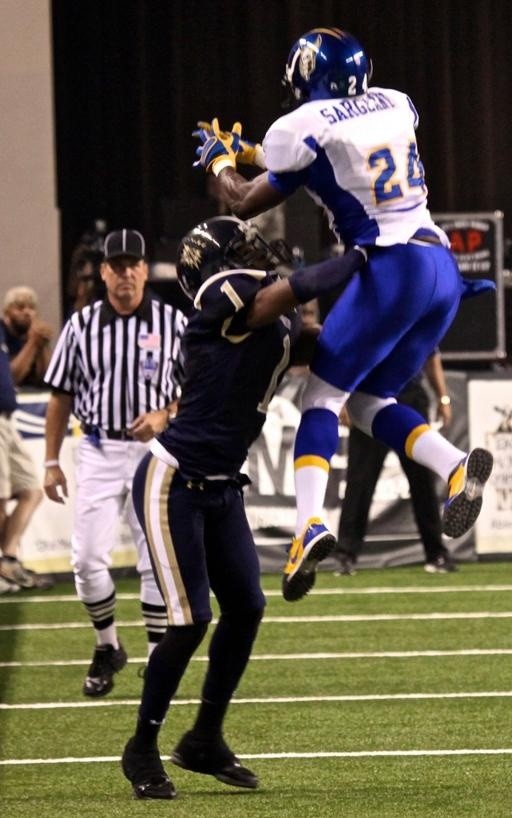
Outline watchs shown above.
[437,395,450,405]
[163,407,176,425]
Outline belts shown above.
[414,235,442,244]
[175,469,252,492]
[80,421,137,441]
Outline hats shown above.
[104,230,144,260]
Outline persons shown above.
[121,213,367,799]
[0,313,46,594]
[43,228,189,699]
[334,343,459,576]
[3,285,55,385]
[192,26,494,603]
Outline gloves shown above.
[193,117,242,178]
[192,121,257,165]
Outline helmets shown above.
[282,27,372,104]
[178,216,306,303]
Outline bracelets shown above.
[253,143,267,170]
[211,159,237,178]
[44,459,60,468]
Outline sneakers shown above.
[0,556,34,590]
[442,449,493,538]
[282,517,336,601]
[0,577,20,595]
[171,730,257,788]
[121,734,177,799]
[424,556,459,573]
[334,553,356,577]
[83,637,127,696]
